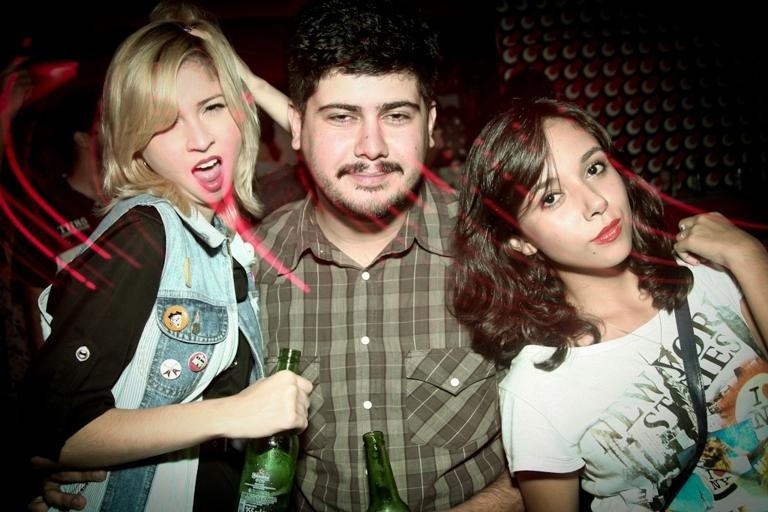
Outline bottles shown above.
[234,346,302,512]
[361,430,411,512]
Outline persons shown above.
[444,96,768,511]
[23,3,314,512]
[14,98,112,352]
[25,1,526,512]
